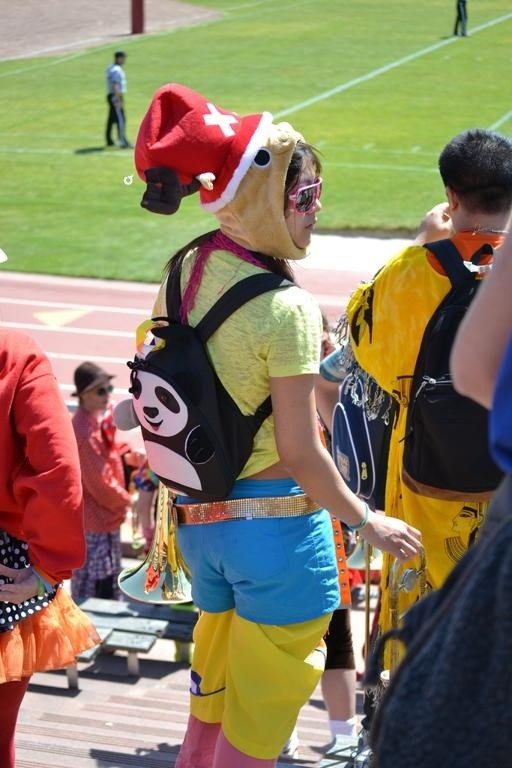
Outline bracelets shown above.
[347,502,371,534]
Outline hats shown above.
[135,84,306,258]
[72,362,112,396]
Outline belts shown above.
[172,494,324,525]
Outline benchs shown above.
[42,599,198,690]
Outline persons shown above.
[451,0,472,39]
[311,276,401,520]
[343,122,510,688]
[137,81,427,767]
[66,357,137,602]
[279,309,361,768]
[371,221,512,768]
[316,311,342,457]
[0,326,100,767]
[104,47,136,150]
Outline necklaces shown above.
[461,220,511,239]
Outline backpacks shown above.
[403,240,503,491]
[126,245,298,502]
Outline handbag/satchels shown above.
[328,369,396,510]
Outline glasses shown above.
[289,177,322,216]
